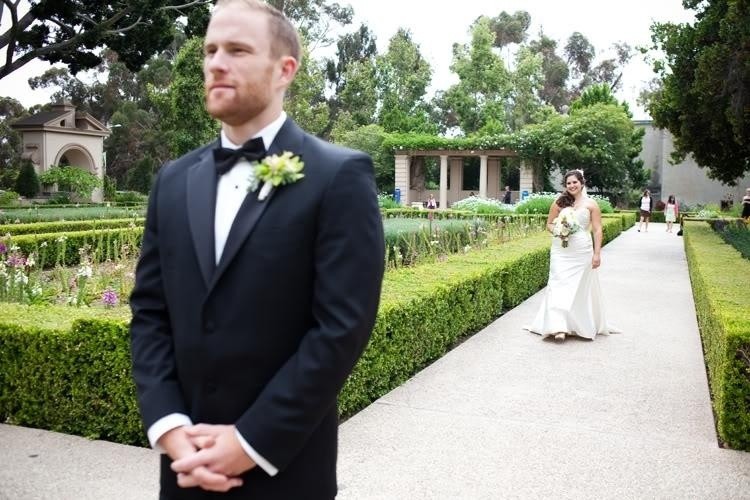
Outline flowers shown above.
[552,206,580,248]
[247,150,306,201]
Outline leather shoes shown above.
[555,333,566,341]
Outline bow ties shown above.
[213,137,266,174]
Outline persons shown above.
[741,188,750,221]
[502,186,512,203]
[664,194,678,232]
[427,194,436,210]
[129,0,386,500]
[469,191,474,196]
[637,189,653,232]
[523,170,621,342]
